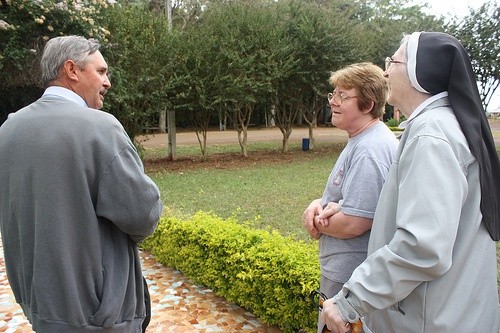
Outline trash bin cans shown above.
[302,138,309,151]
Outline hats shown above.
[408,31,500,242]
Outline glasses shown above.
[328,93,360,106]
[384,56,406,71]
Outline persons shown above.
[0,35,163,333]
[301,62,400,333]
[323,33,500,333]
